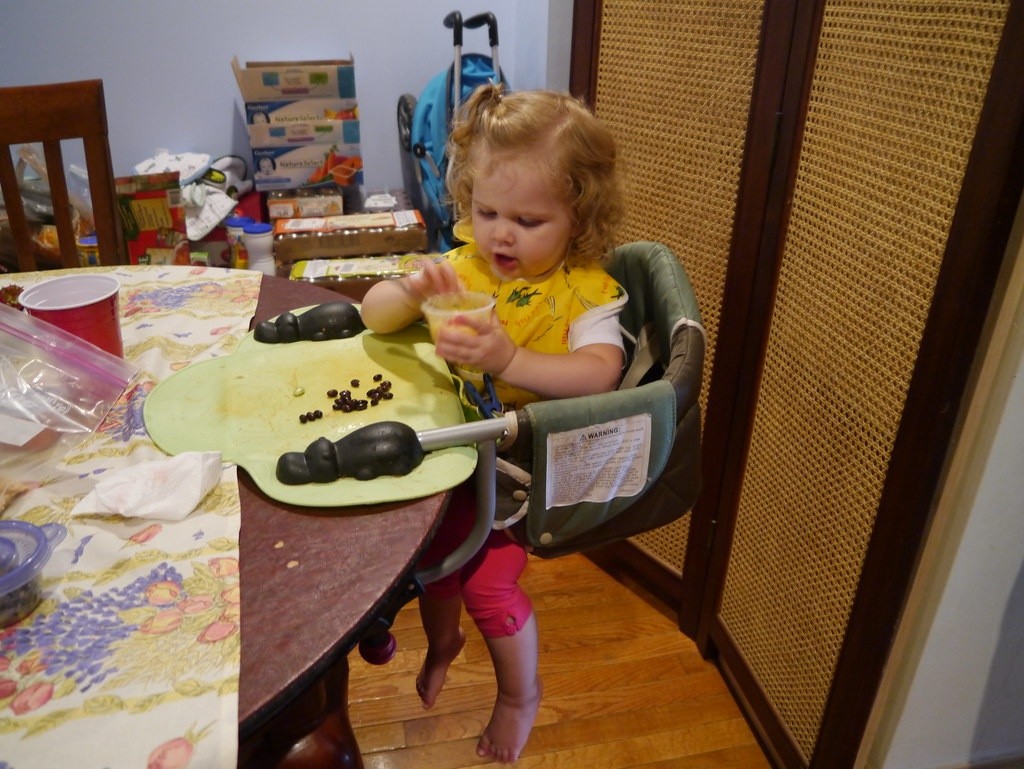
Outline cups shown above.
[17,274,123,405]
[421,291,495,347]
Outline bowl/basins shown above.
[0,520,67,628]
[330,165,356,186]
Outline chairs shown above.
[485,241,704,559]
[0,79,130,272]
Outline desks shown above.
[0,264,453,769]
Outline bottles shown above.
[244,224,276,277]
[224,217,254,270]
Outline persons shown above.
[360,84,631,764]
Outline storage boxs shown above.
[265,185,344,222]
[252,148,364,192]
[274,209,428,266]
[289,255,441,302]
[230,54,361,148]
